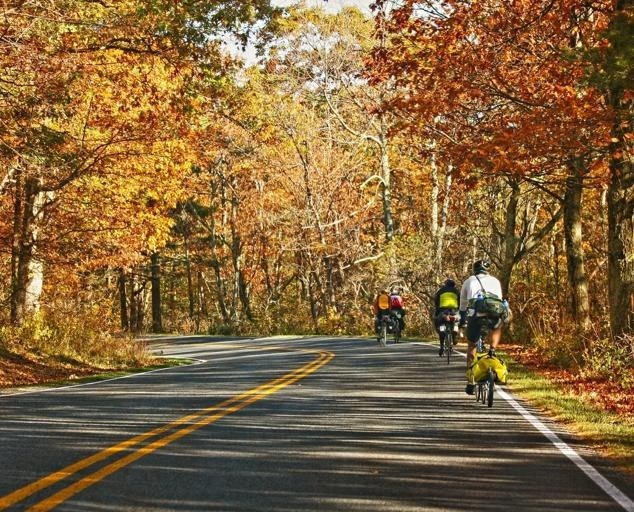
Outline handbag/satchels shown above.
[479,293,506,319]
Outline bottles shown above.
[504,299,509,309]
[477,294,484,300]
[477,336,482,353]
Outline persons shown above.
[372,288,391,340]
[388,289,406,327]
[460,259,503,395]
[435,277,460,346]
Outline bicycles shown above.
[374,312,404,348]
[459,317,501,408]
[440,310,459,365]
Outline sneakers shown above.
[463,381,476,395]
[488,349,497,356]
[438,346,446,356]
[452,333,459,345]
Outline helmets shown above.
[444,279,457,288]
[472,259,491,273]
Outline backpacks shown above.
[439,290,459,310]
[390,295,402,309]
[379,293,389,310]
